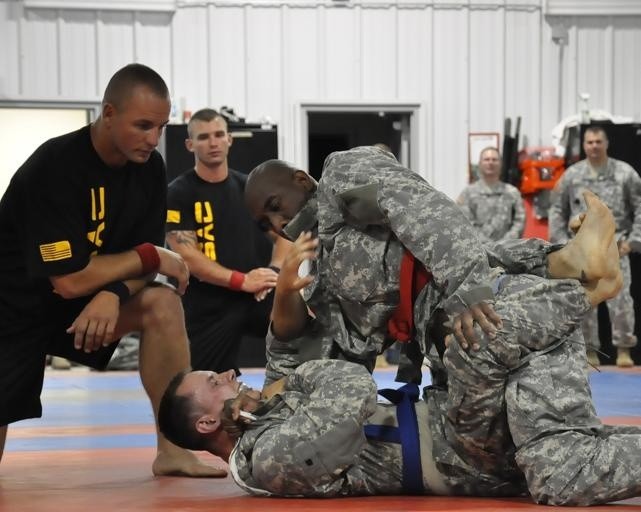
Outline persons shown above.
[166,110,296,370]
[456,147,524,242]
[158,145,640,507]
[547,125,640,367]
[1,64,229,508]
